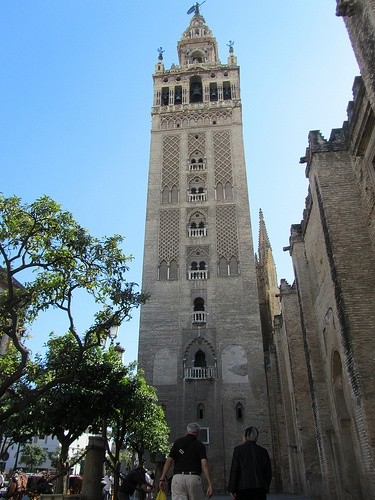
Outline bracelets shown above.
[159,480,164,481]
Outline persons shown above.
[160,424,212,500]
[227,427,272,500]
[0,466,173,500]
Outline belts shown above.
[174,471,200,474]
